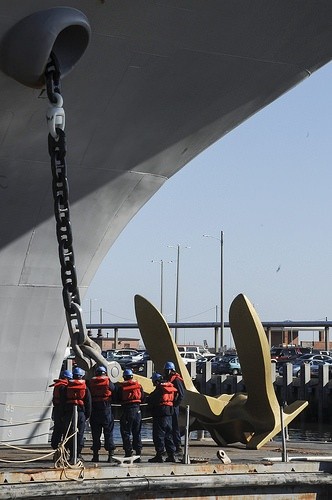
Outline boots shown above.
[92,449,100,462]
[148,451,163,463]
[134,450,141,462]
[77,448,84,463]
[175,444,183,456]
[165,452,176,462]
[108,450,117,462]
[67,449,74,464]
[123,450,132,464]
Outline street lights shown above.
[85,298,97,331]
[166,243,191,345]
[151,259,173,315]
[203,230,224,357]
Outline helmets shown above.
[124,370,133,376]
[73,367,83,375]
[152,374,161,383]
[62,370,73,379]
[96,366,107,373]
[164,362,175,370]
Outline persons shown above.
[88,365,117,462]
[113,369,145,457]
[49,368,92,463]
[146,362,186,462]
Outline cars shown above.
[62,345,332,379]
